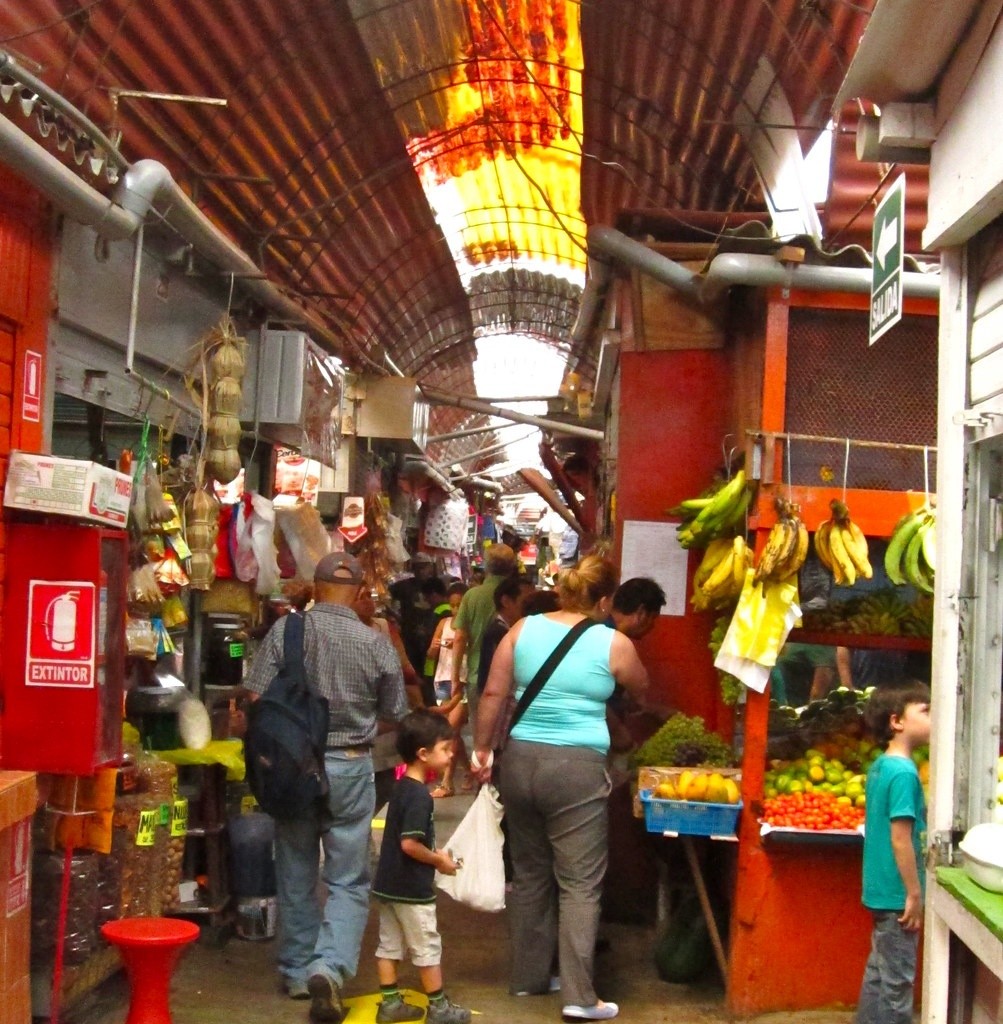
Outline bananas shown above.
[665,470,934,641]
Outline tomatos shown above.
[761,790,864,830]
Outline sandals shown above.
[461,770,473,789]
[430,783,454,798]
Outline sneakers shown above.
[425,999,471,1024]
[375,993,424,1024]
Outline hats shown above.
[313,551,363,585]
[411,553,431,566]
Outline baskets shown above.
[638,788,741,835]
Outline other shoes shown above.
[562,1001,618,1018]
[513,976,563,997]
[307,973,342,1024]
[284,975,310,997]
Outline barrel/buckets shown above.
[229,887,277,942]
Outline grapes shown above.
[709,615,742,706]
[627,711,742,770]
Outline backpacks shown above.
[242,610,329,820]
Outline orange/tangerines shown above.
[766,684,933,807]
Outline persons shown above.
[371,708,472,1024]
[273,576,314,617]
[807,645,838,702]
[451,544,517,703]
[388,567,475,797]
[853,678,931,1024]
[491,590,562,893]
[477,573,537,698]
[837,646,908,691]
[242,551,410,1024]
[470,556,650,1019]
[594,576,668,951]
[347,580,427,817]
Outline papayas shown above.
[653,768,739,805]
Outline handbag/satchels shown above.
[431,780,508,911]
